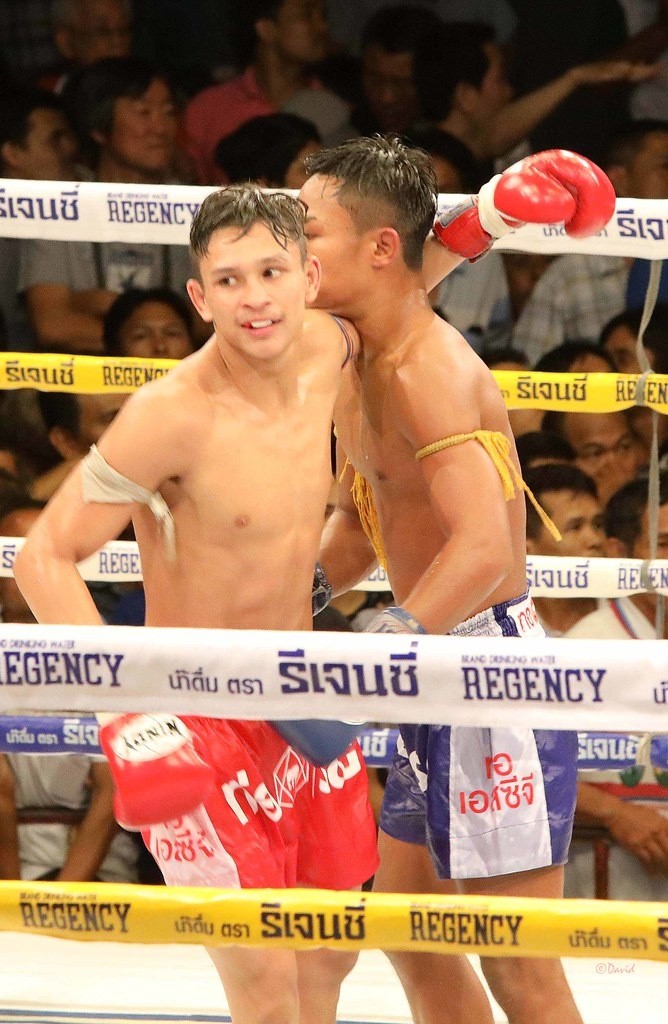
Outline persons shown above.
[14,146,615,1024]
[298,131,587,1024]
[0,0,668,883]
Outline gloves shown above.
[269,609,425,767]
[431,150,615,264]
[96,706,212,831]
